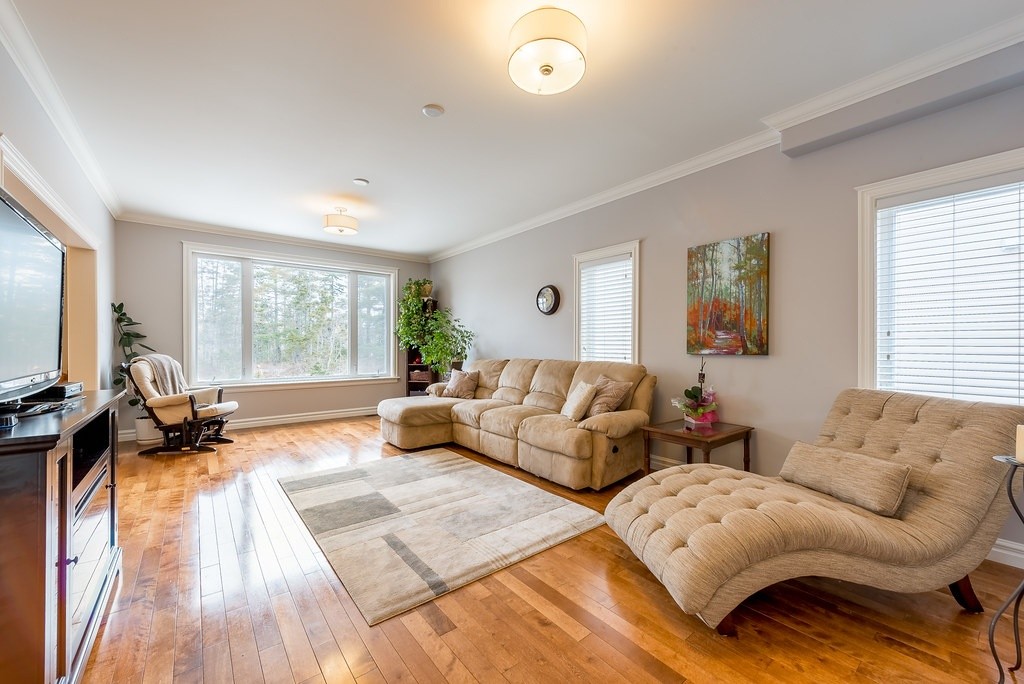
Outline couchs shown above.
[603,386,1024,638]
[377,358,657,491]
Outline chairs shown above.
[121,354,239,456]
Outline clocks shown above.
[536,284,560,315]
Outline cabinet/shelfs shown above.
[405,299,438,396]
[0,409,123,684]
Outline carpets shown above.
[277,447,607,627]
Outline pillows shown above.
[779,440,912,517]
[440,368,480,399]
[585,374,634,417]
[561,381,597,422]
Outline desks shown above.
[987,456,1024,684]
[640,418,755,478]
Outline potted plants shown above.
[418,306,475,375]
[393,278,434,351]
[111,302,165,445]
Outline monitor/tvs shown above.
[0,185,66,415]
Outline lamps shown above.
[322,214,359,235]
[506,7,589,96]
